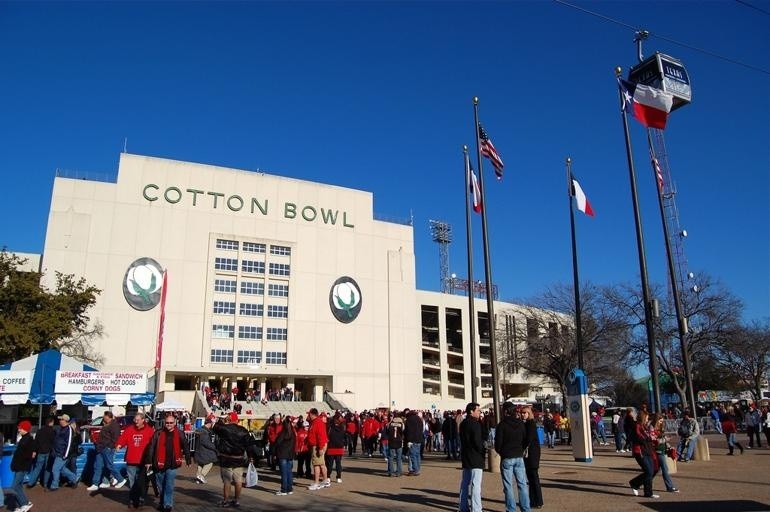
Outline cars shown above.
[80,416,155,434]
[599,407,638,424]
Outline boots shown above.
[726,446,733,456]
[735,442,743,454]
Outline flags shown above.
[152,270,170,369]
[618,76,674,131]
[468,159,482,216]
[479,118,506,182]
[568,173,596,217]
[647,134,664,195]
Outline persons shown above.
[204,385,301,412]
[343,400,612,511]
[612,399,770,498]
[0,411,349,512]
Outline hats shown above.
[58,414,70,422]
[17,420,32,432]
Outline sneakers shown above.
[669,487,680,493]
[652,493,660,499]
[389,469,421,476]
[633,487,639,496]
[16,477,126,512]
[196,474,342,507]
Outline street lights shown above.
[665,230,704,428]
[478,280,483,299]
[429,220,452,293]
[451,273,457,294]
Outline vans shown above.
[150,405,197,422]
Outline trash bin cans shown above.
[0,445,18,489]
[537,427,544,445]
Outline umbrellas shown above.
[0,351,157,430]
